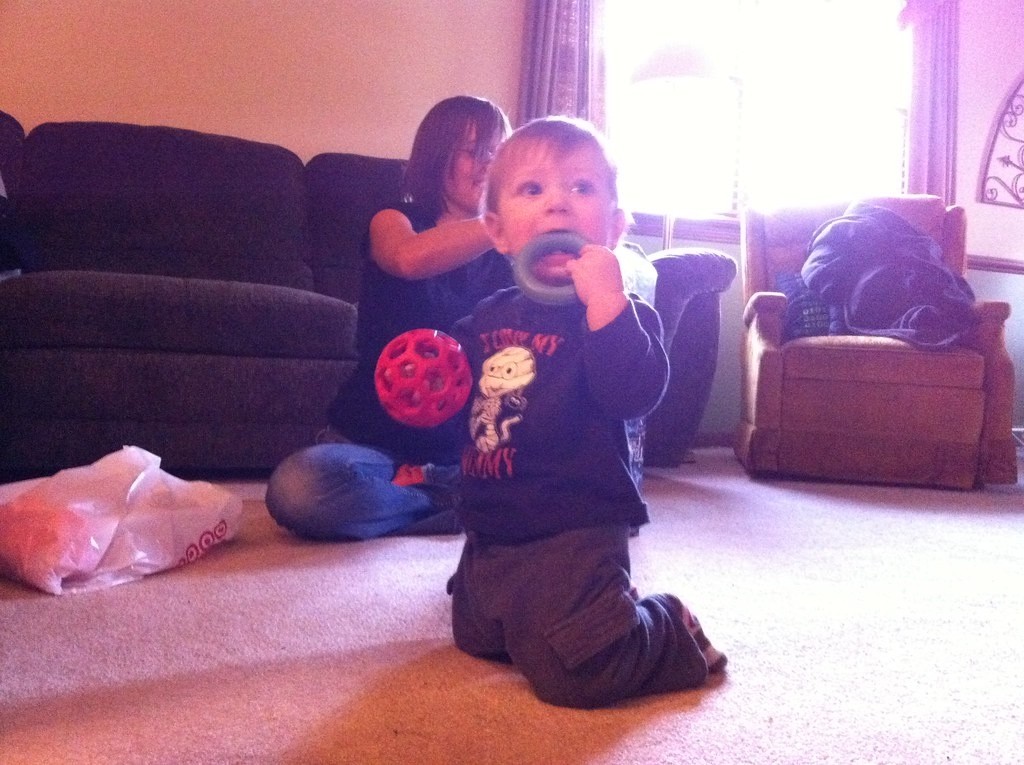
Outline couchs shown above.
[0,112,736,470]
[734,193,1017,488]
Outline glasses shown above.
[458,148,500,166]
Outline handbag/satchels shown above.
[0,445,244,597]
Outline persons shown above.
[265,95,511,541]
[386,117,728,706]
